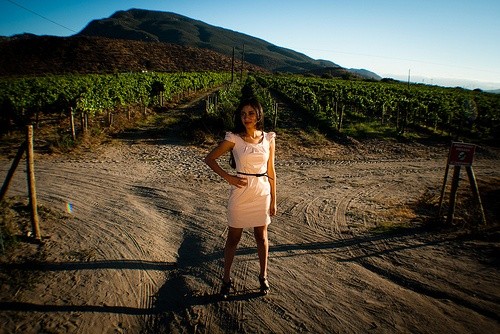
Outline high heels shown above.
[258,274,270,295]
[221,278,232,299]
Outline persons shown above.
[204,97,277,296]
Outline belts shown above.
[236,171,273,180]
